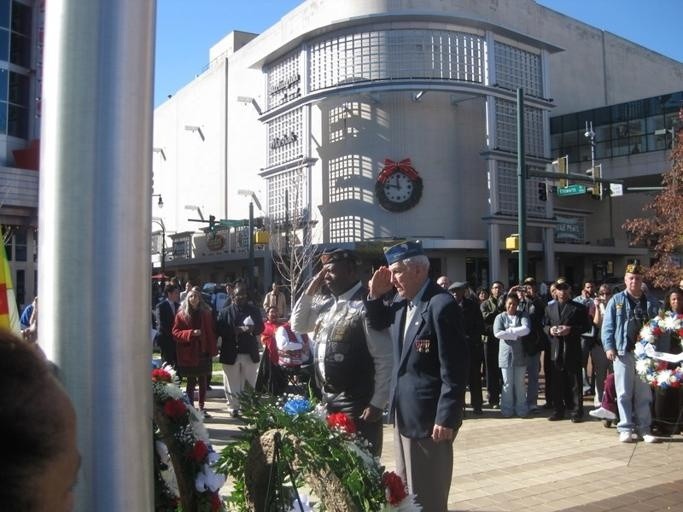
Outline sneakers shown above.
[472,401,657,443]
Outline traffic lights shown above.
[585,164,599,199]
[537,182,545,201]
[208,215,215,230]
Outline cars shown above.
[598,276,648,299]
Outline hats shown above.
[383,240,423,264]
[320,248,352,264]
[449,265,642,291]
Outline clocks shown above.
[375,158,422,213]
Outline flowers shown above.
[152,357,228,512]
[634,308,683,389]
[211,386,419,512]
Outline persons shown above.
[601,263,661,443]
[289,247,394,460]
[362,241,467,512]
[493,292,531,417]
[509,276,626,422]
[436,276,505,414]
[152,276,316,420]
[655,288,683,431]
[0,328,82,512]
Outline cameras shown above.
[518,285,527,292]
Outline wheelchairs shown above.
[600,391,618,428]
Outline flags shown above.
[0,232,22,341]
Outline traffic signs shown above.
[558,184,585,196]
[610,183,622,197]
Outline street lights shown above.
[151,194,163,208]
